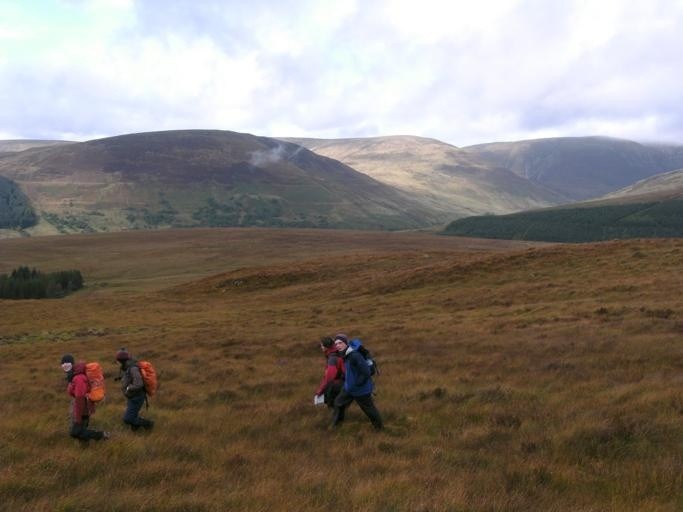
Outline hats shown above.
[117,352,128,360]
[322,334,347,347]
[61,355,74,364]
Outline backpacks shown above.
[350,338,376,376]
[72,363,105,401]
[129,361,157,396]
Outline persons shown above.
[330,334,384,431]
[60,354,111,442]
[115,346,154,432]
[314,337,345,416]
